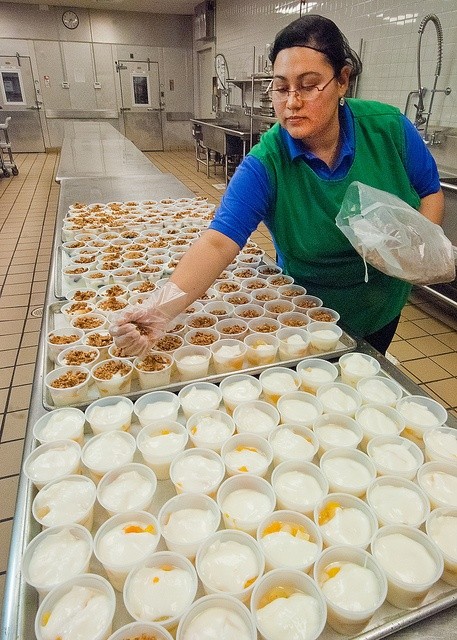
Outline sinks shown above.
[190,117,262,157]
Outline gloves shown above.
[107,281,187,356]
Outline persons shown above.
[108,14,446,360]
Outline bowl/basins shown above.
[338,352,381,389]
[276,389,325,430]
[32,572,117,640]
[365,474,431,531]
[195,526,267,603]
[22,438,82,492]
[107,342,136,360]
[82,395,134,436]
[217,248,281,338]
[220,432,274,480]
[266,422,320,467]
[208,338,248,377]
[80,428,137,477]
[421,426,457,463]
[296,358,338,394]
[312,412,364,454]
[134,391,181,428]
[106,620,172,640]
[316,381,362,419]
[250,565,329,639]
[60,300,96,319]
[365,433,425,481]
[56,196,216,285]
[81,329,113,349]
[65,288,97,300]
[276,326,312,362]
[19,521,94,599]
[219,373,263,409]
[177,381,222,420]
[126,280,157,299]
[122,550,199,630]
[175,592,255,640]
[186,408,236,455]
[90,357,134,398]
[306,320,343,355]
[69,312,106,335]
[31,473,99,534]
[94,461,158,518]
[132,351,174,391]
[127,293,152,307]
[311,543,389,636]
[32,407,86,447]
[356,376,404,408]
[281,275,342,329]
[269,460,329,512]
[396,395,448,434]
[354,402,405,441]
[369,524,446,612]
[258,366,302,402]
[149,287,217,351]
[243,332,281,369]
[426,508,457,588]
[137,419,190,481]
[96,296,130,314]
[44,366,91,407]
[319,446,378,498]
[168,446,226,500]
[92,510,162,594]
[232,400,282,435]
[415,463,457,509]
[312,494,381,551]
[172,344,213,383]
[45,327,85,361]
[98,283,130,299]
[157,492,223,562]
[57,345,101,369]
[216,473,277,539]
[256,508,321,572]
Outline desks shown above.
[0,177,457,640]
[54,140,163,184]
[63,120,130,139]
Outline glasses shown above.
[265,76,335,102]
